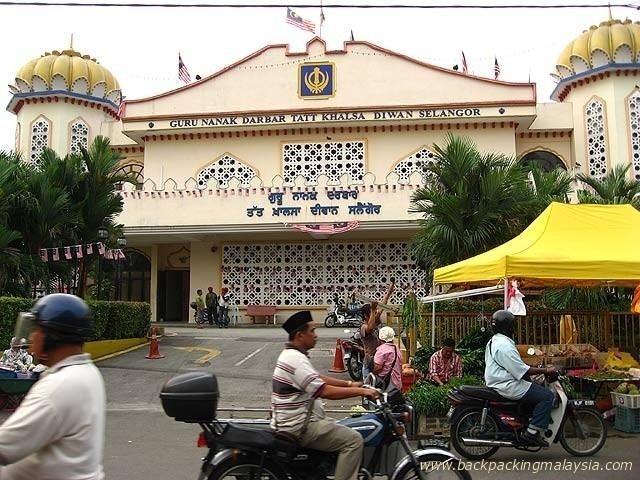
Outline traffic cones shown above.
[144,326,167,360]
[325,336,348,375]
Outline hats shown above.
[282,311,313,332]
[361,304,377,315]
[379,327,395,342]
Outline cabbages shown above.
[616,382,639,395]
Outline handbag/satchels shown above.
[372,375,390,390]
[269,436,299,464]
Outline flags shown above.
[495,58,500,79]
[320,9,325,25]
[178,57,191,86]
[462,52,469,75]
[286,8,317,34]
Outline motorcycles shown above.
[444,346,609,461]
[322,298,371,329]
[157,369,472,480]
[190,295,231,324]
[341,332,364,381]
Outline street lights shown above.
[95,223,111,299]
[116,235,128,300]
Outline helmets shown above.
[492,310,517,334]
[23,293,95,336]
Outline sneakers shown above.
[522,430,549,447]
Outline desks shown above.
[575,375,640,402]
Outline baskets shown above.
[610,391,640,433]
[516,343,600,368]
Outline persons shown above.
[360,282,393,401]
[217,287,229,328]
[205,286,218,324]
[483,310,556,448]
[0,337,33,373]
[372,325,404,394]
[196,289,205,329]
[0,293,106,479]
[425,339,464,386]
[270,310,381,480]
